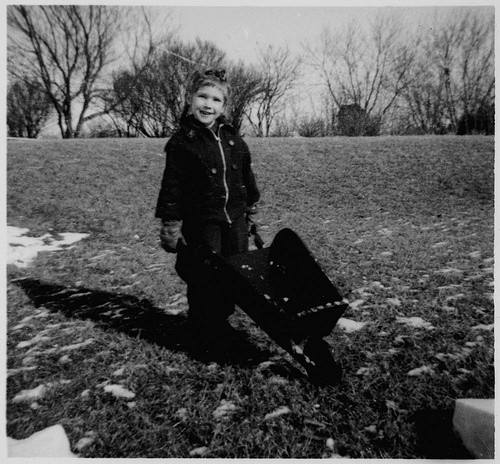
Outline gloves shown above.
[160,220,188,255]
[246,204,259,238]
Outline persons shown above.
[150,66,261,358]
